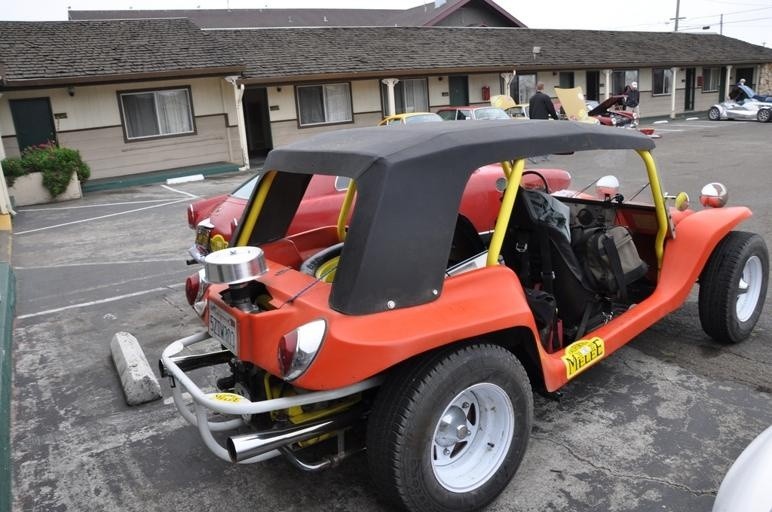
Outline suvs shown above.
[158,118,770,512]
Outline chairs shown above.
[500,188,604,336]
[453,211,558,352]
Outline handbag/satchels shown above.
[571,223,651,302]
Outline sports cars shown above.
[708,97,772,121]
[185,164,570,265]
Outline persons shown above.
[731,77,747,101]
[528,82,559,121]
[624,81,640,124]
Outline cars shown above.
[377,111,443,126]
[489,86,603,125]
[436,104,512,120]
[547,94,666,140]
[737,84,772,102]
